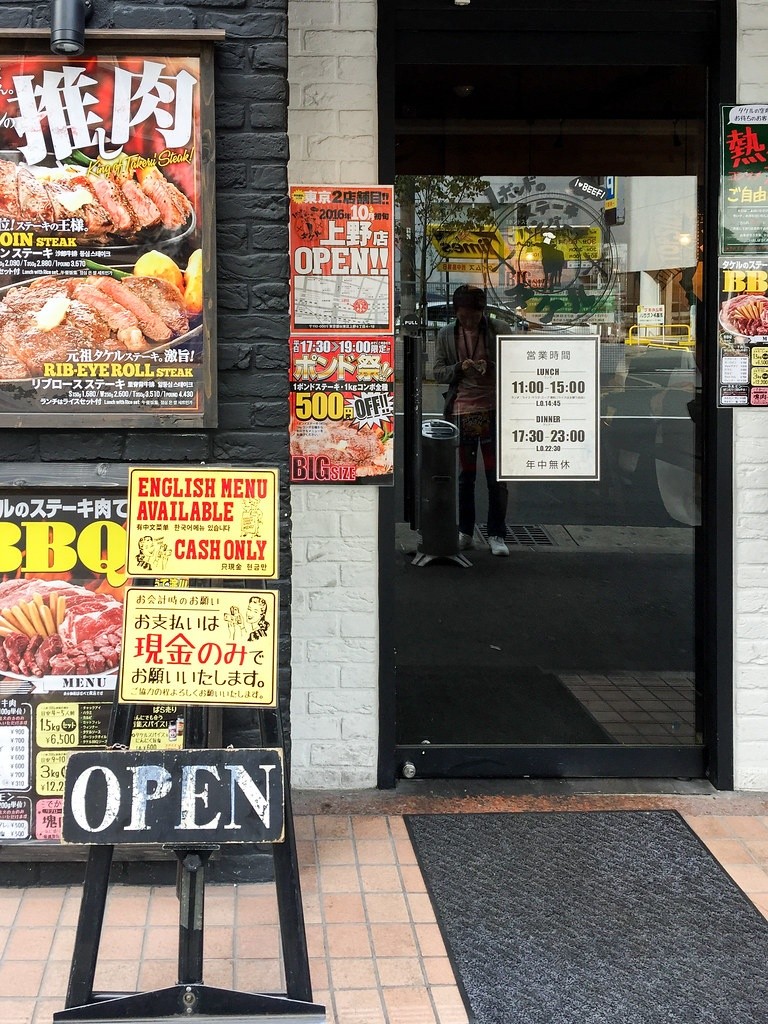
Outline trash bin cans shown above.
[405,418,475,568]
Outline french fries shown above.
[0,591,67,640]
[733,300,764,319]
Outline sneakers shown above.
[488,535,509,556]
[458,532,475,550]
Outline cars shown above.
[394,300,577,382]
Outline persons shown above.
[434,285,516,555]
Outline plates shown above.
[0,261,206,387]
[0,203,198,270]
[2,650,125,686]
[719,311,768,340]
